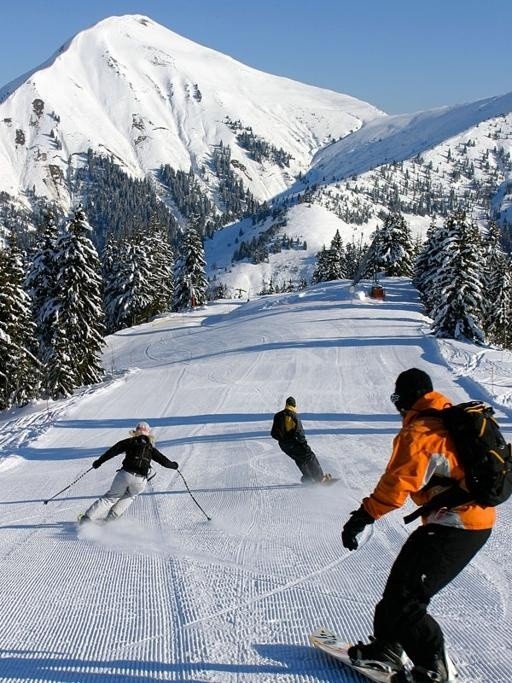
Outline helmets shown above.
[136,422,151,435]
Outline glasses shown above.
[389,390,405,404]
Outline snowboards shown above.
[311,628,459,677]
[299,473,338,487]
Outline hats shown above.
[286,397,296,407]
[395,368,434,410]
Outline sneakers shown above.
[348,638,403,671]
[390,654,448,683]
[318,474,331,486]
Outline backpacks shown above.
[416,400,512,507]
[128,437,154,476]
[271,410,295,442]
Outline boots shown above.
[80,510,118,526]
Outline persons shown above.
[82,420,180,525]
[341,367,511,683]
[270,395,332,487]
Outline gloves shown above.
[342,504,374,551]
